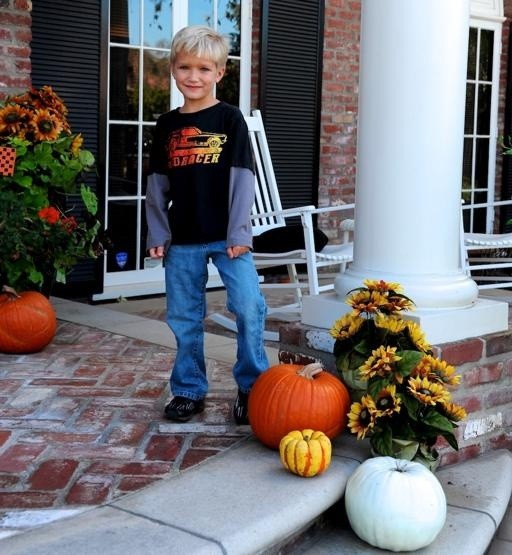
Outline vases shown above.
[370,434,419,464]
[342,367,372,392]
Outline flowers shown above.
[336,280,429,356]
[347,343,468,448]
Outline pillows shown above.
[252,226,329,275]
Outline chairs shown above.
[209,110,359,342]
[464,232,512,289]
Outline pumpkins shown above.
[281,426,333,475]
[249,364,349,449]
[345,457,448,551]
[0,288,55,355]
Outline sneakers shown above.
[165,395,205,419]
[234,391,251,425]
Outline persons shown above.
[145,24,267,425]
